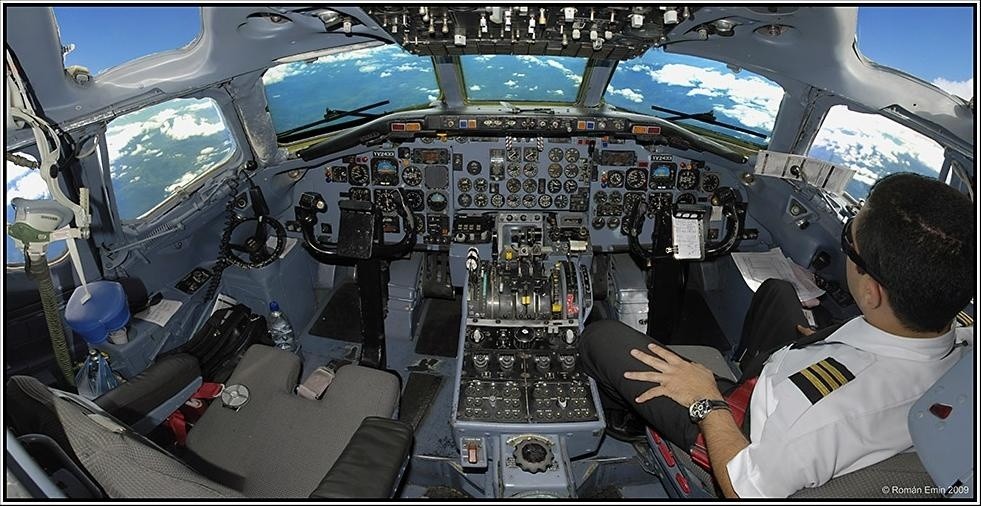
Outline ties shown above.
[736,316,856,385]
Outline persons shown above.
[576,171,976,499]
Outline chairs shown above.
[639,345,974,499]
[5,344,414,499]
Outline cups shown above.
[109,327,130,347]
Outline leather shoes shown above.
[602,407,648,443]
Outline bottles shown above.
[85,351,104,379]
[262,302,297,355]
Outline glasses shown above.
[840,217,886,287]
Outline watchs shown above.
[689,398,732,423]
